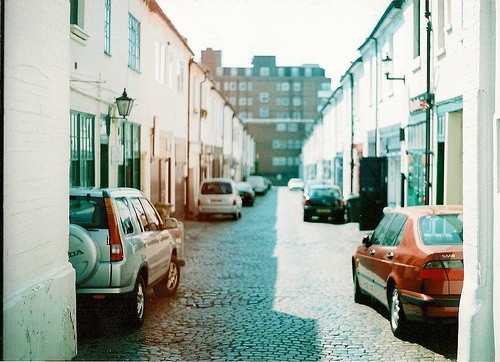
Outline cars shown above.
[352,206,463,340]
[304,184,346,224]
[237,182,255,205]
[198,178,242,221]
[288,178,305,191]
[246,176,269,193]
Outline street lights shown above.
[113,89,135,186]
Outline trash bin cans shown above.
[347,195,360,222]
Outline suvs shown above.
[69,184,186,326]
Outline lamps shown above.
[382,55,406,83]
[104,88,135,119]
